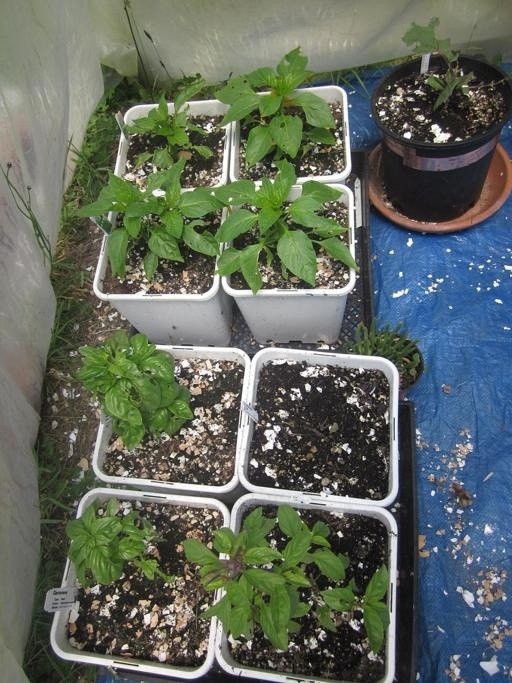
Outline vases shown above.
[236,347,399,508]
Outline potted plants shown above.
[75,161,227,345]
[185,491,398,683]
[214,155,356,343]
[100,70,230,184]
[217,44,350,179]
[49,485,228,678]
[75,330,243,489]
[369,17,500,218]
